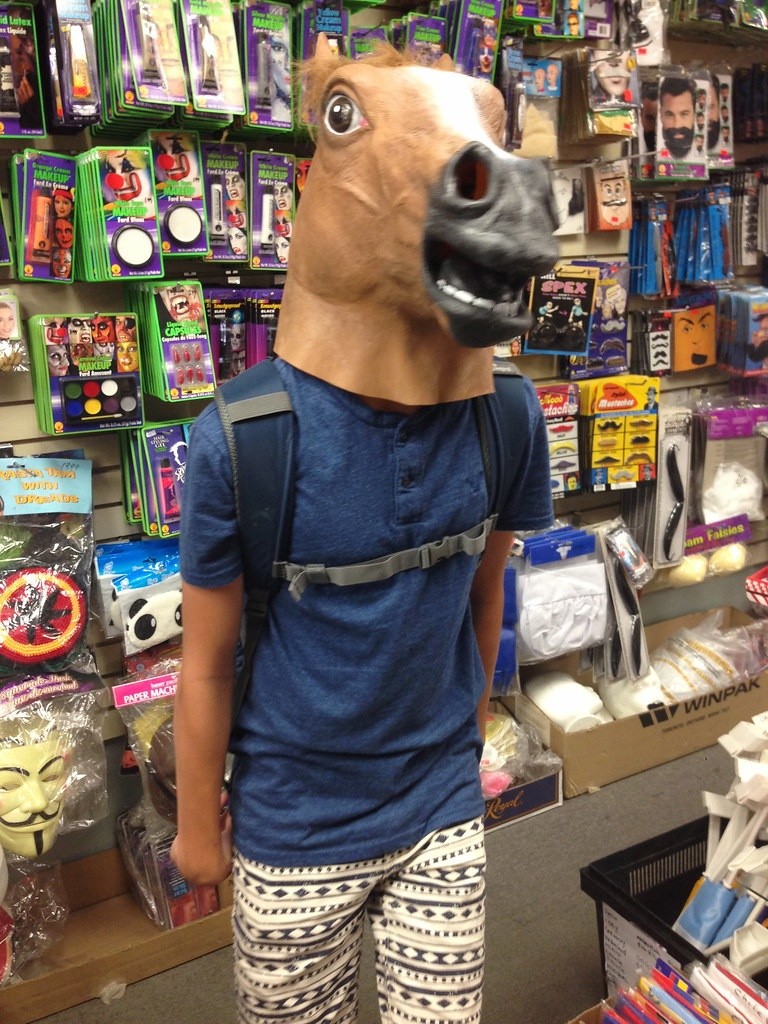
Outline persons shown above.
[273,181,293,263]
[536,301,558,323]
[641,81,659,159]
[693,72,719,150]
[53,188,74,278]
[105,150,151,202]
[659,77,695,157]
[568,298,588,326]
[225,171,249,254]
[169,31,555,1024]
[696,89,706,152]
[720,83,730,144]
[44,315,139,376]
[156,132,199,182]
